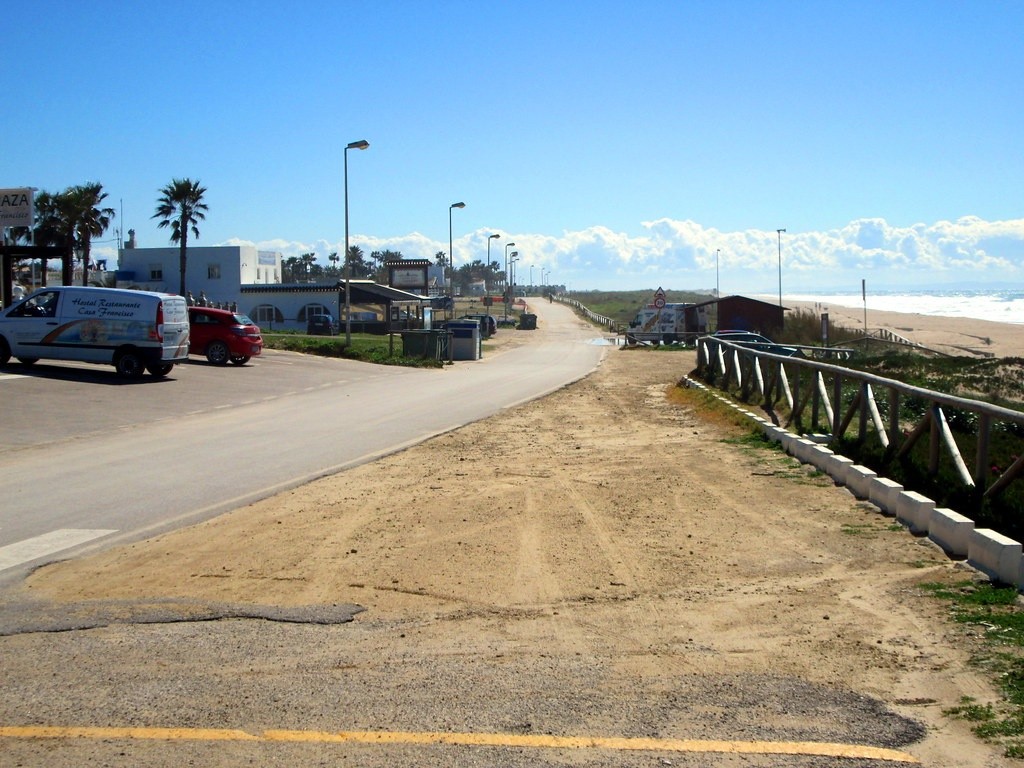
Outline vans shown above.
[627,301,701,347]
[0,285,191,380]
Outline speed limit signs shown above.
[656,299,665,309]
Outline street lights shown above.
[448,201,466,320]
[777,228,787,306]
[344,140,370,348]
[717,249,721,299]
[510,250,520,312]
[541,267,545,285]
[486,234,501,337]
[504,242,515,323]
[529,265,534,287]
[547,271,551,284]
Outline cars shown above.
[306,314,341,335]
[715,329,766,349]
[392,309,423,321]
[189,306,264,365]
[457,314,498,339]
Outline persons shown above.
[199,291,207,307]
[49,293,58,317]
[550,294,552,303]
[186,291,196,307]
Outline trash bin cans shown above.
[484,296,492,306]
[519,314,537,330]
[399,328,447,361]
